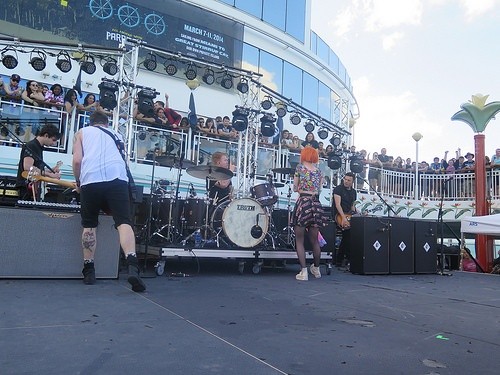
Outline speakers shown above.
[350,215,390,274]
[381,217,415,275]
[412,220,440,275]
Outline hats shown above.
[465,153,474,158]
[10,74,20,81]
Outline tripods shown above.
[147,163,231,249]
[268,174,297,252]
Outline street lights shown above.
[411,132,424,201]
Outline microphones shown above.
[443,176,454,184]
[356,176,366,180]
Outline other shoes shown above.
[296,267,308,281]
[309,263,322,279]
[82,260,95,285]
[126,264,147,292]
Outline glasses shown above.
[33,84,38,87]
[12,79,19,83]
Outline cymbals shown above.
[272,167,296,174]
[186,164,233,180]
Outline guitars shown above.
[21,166,77,190]
[335,211,369,229]
[28,160,63,201]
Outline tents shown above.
[459,214,500,270]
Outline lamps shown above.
[261,99,272,110]
[0,49,18,69]
[276,107,287,119]
[55,53,72,73]
[317,127,329,139]
[102,58,118,76]
[164,63,177,76]
[237,78,249,93]
[303,119,316,132]
[79,56,96,74]
[202,69,215,85]
[184,64,197,81]
[221,73,233,89]
[290,113,302,126]
[330,134,342,146]
[143,53,156,70]
[30,51,47,71]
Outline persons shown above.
[0,74,240,174]
[332,173,357,269]
[253,129,366,194]
[204,153,235,231]
[292,146,325,280]
[492,250,500,274]
[71,112,149,291]
[367,148,500,200]
[460,248,479,272]
[15,124,74,206]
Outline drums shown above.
[144,196,184,234]
[182,197,207,228]
[251,183,277,205]
[222,197,270,248]
